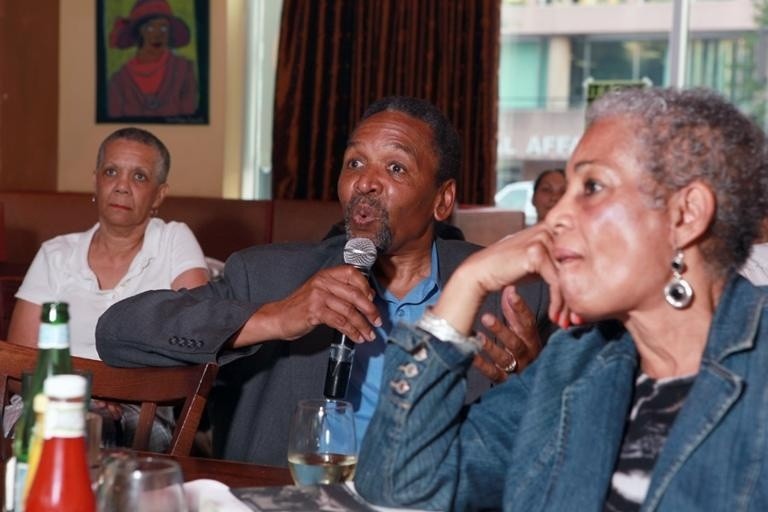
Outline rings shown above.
[492,351,517,373]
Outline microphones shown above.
[323,238,378,400]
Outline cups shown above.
[287,398,359,487]
[98,457,189,512]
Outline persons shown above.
[5,126,211,454]
[95,96,542,467]
[529,164,564,224]
[322,210,466,242]
[349,86,768,512]
[104,1,200,116]
[738,218,767,285]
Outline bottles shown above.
[12,300,97,512]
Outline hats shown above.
[110,1,191,49]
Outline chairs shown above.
[1,339,219,459]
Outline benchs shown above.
[1,186,531,346]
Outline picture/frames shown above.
[95,0,210,127]
